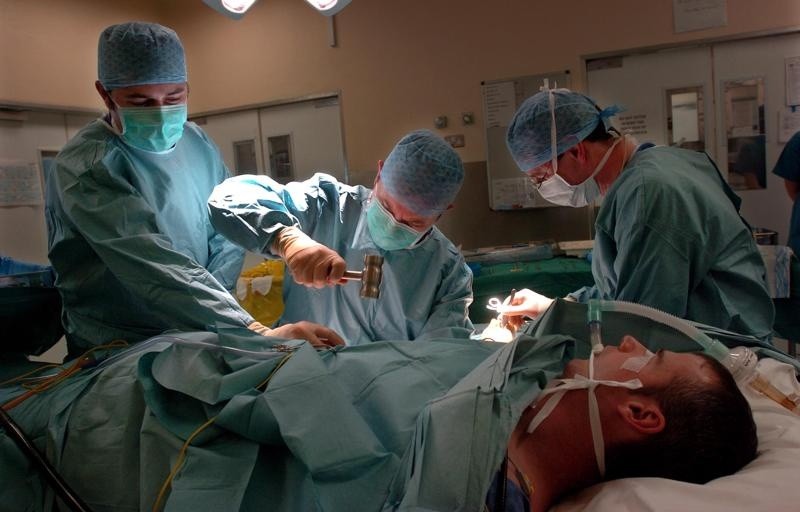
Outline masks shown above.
[367,197,422,250]
[535,174,601,209]
[116,102,187,153]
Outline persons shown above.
[772,129,800,261]
[491,85,790,354]
[485,334,757,512]
[42,20,352,369]
[206,127,480,342]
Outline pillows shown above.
[552,357,800,511]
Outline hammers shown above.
[341,254,387,298]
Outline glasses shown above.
[528,162,551,191]
